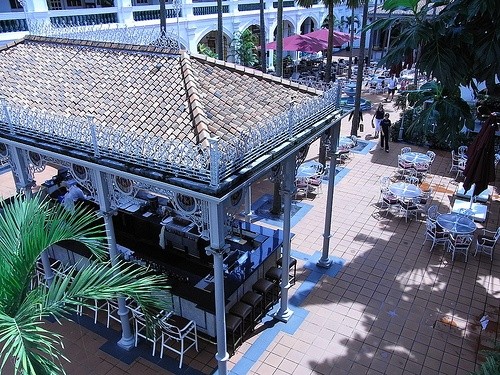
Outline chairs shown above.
[266,56,500,262]
[28,255,199,369]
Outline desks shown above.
[389,182,421,214]
[436,214,477,253]
[402,152,431,176]
[294,166,316,196]
[338,138,354,159]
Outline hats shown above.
[385,113,389,116]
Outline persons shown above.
[399,78,409,90]
[378,113,392,153]
[383,74,398,101]
[371,103,386,138]
[348,109,363,131]
[281,54,347,82]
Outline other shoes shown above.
[374,134,379,139]
[384,99,387,102]
[385,150,389,153]
[381,147,384,150]
[391,98,393,101]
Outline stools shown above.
[226,256,297,355]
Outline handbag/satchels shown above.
[360,124,363,132]
[372,119,375,128]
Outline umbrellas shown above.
[256,34,335,73]
[303,27,364,57]
[461,111,500,211]
